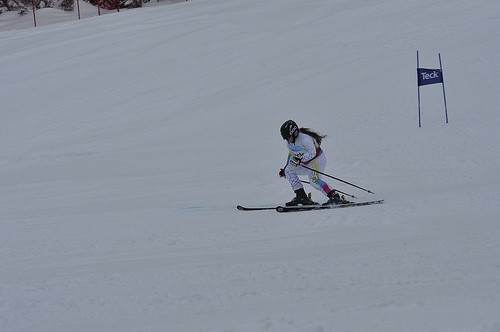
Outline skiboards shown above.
[237,200,384,212]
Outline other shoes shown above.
[285,188,308,206]
[321,189,340,206]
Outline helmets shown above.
[280,120,298,140]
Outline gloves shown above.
[279,168,285,177]
[290,157,302,167]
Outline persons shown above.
[280,120,340,206]
